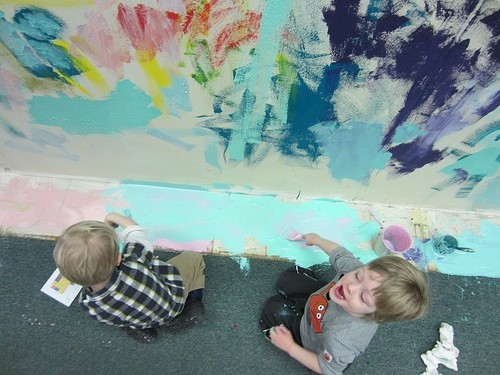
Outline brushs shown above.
[286,230,305,243]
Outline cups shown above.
[433,234,458,256]
[374,225,412,257]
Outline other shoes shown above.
[121,326,157,344]
[165,299,206,336]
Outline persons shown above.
[53,213,205,342]
[259,233,427,375]
